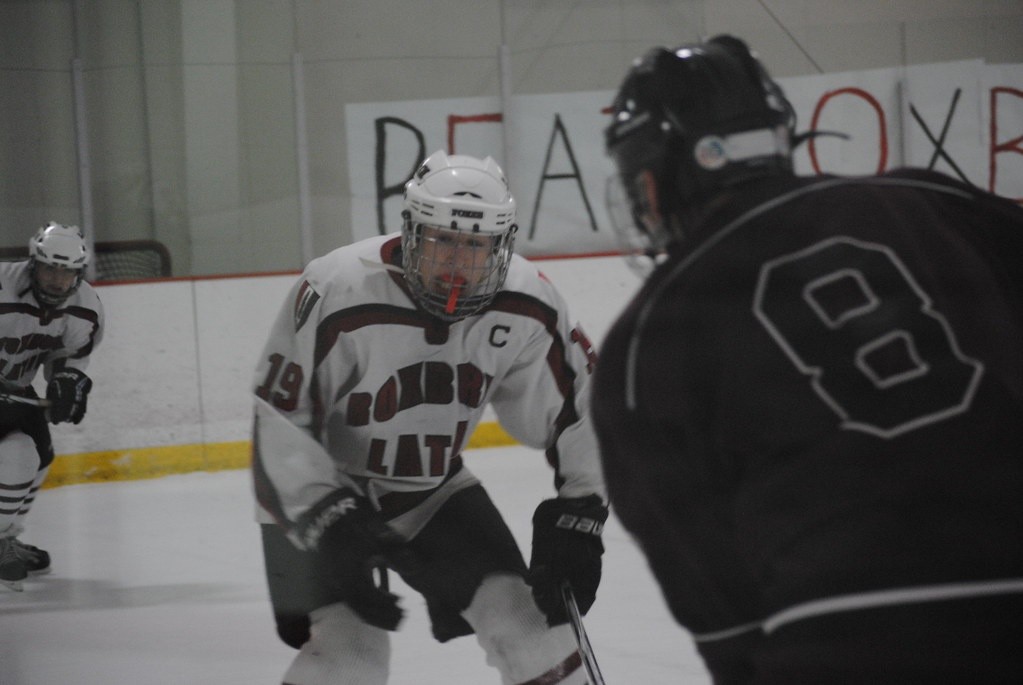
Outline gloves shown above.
[526,494,607,628]
[46,366,91,425]
[317,507,443,631]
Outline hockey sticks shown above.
[0,392,50,411]
[560,577,606,685]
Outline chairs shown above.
[0,233,180,287]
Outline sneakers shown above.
[11,524,52,575]
[0,533,28,592]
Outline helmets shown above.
[606,33,796,279]
[29,221,89,269]
[401,149,516,321]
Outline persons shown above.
[590,35,1023,685]
[250,150,611,685]
[0,219,105,593]
